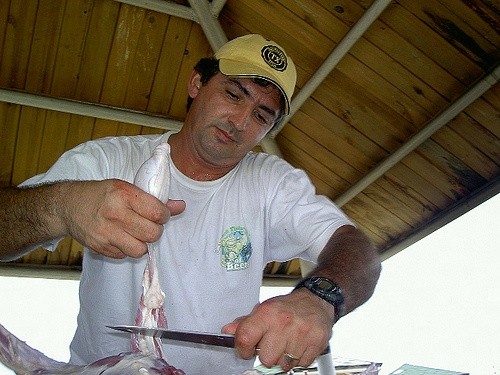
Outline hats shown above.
[212,33,297,116]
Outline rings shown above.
[283,351,298,363]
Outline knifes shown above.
[105,324,331,356]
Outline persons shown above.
[0,34,381,375]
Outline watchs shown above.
[293,276,343,324]
